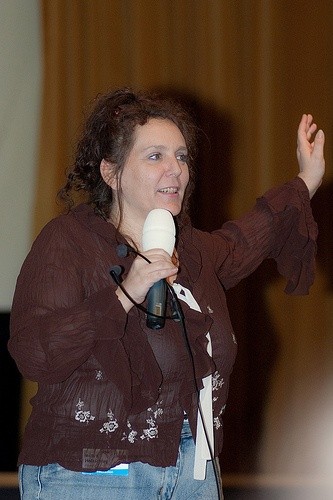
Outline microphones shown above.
[141,209,176,327]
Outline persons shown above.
[9,88,326,499]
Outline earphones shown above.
[110,265,121,286]
[116,244,130,259]
